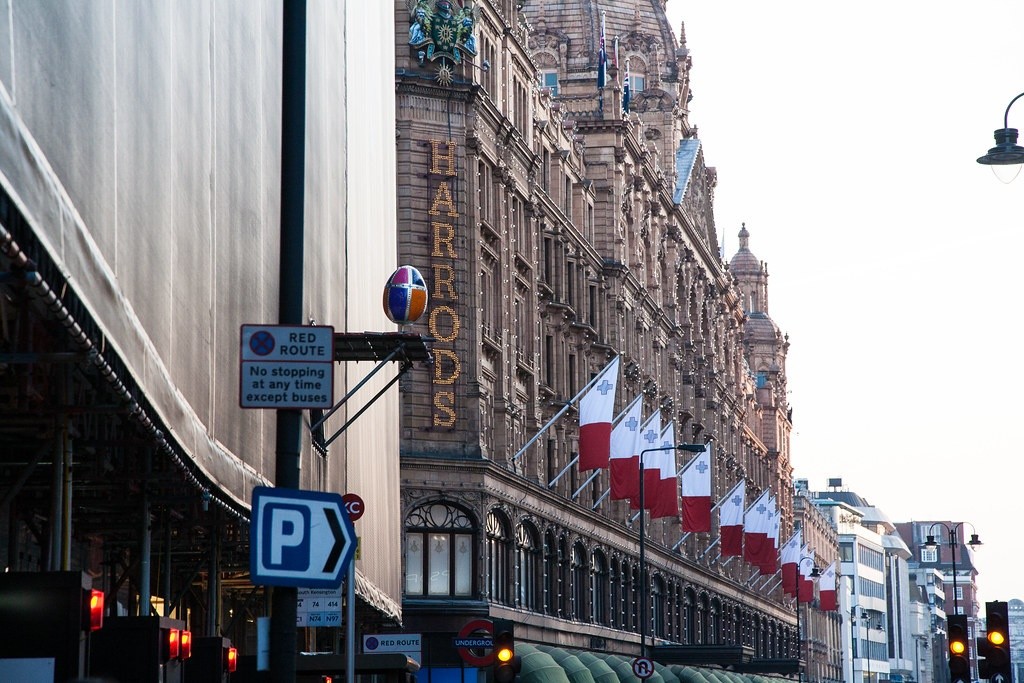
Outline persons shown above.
[457,7,477,55]
[408,0,433,45]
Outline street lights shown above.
[795,557,824,683]
[867,616,884,683]
[916,636,930,683]
[639,444,707,683]
[850,604,868,683]
[923,521,984,614]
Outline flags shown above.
[627,415,663,509]
[609,398,642,500]
[652,421,677,516]
[580,360,622,472]
[680,441,835,611]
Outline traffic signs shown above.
[251,486,359,588]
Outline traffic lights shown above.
[977,602,1012,683]
[492,620,515,683]
[323,675,334,683]
[947,615,971,683]
